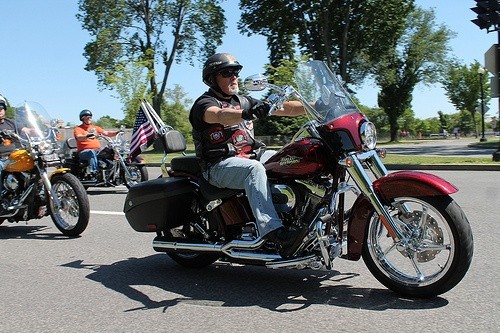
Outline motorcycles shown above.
[123,57,475,299]
[60,123,149,190]
[0,99,90,238]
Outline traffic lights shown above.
[469,0,500,34]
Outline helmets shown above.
[203,53,244,81]
[80,110,92,121]
[0,96,8,111]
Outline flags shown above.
[130,99,166,161]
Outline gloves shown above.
[242,100,272,121]
[116,130,124,135]
[315,99,333,112]
[87,133,95,139]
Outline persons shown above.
[0,95,57,206]
[190,52,330,248]
[74,110,124,179]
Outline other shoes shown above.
[263,226,299,241]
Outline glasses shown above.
[0,105,5,109]
[218,69,239,78]
[84,115,92,119]
